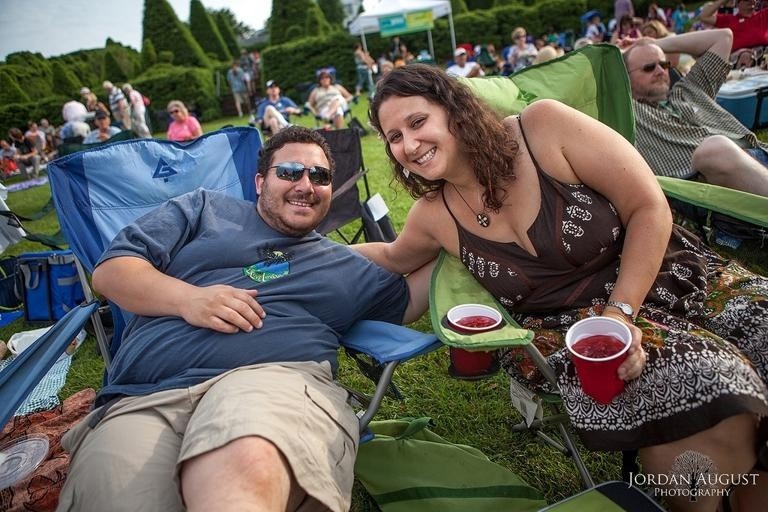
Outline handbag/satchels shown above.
[21,254,84,322]
[142,95,150,106]
[353,417,546,510]
[0,254,24,310]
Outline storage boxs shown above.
[718,70,768,131]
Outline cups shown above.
[564,316,632,404]
[446,303,502,375]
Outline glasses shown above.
[629,61,671,75]
[265,162,332,186]
[170,109,179,115]
[514,35,526,40]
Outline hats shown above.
[532,46,564,65]
[266,79,277,87]
[94,110,106,119]
[455,47,466,57]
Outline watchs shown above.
[605,300,635,324]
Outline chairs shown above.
[0,124,440,443]
[311,127,399,242]
[429,41,768,489]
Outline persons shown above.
[339,66,767,512]
[226,49,260,117]
[166,100,202,142]
[249,79,300,136]
[351,34,432,101]
[0,79,155,182]
[50,124,440,512]
[615,28,768,197]
[444,1,697,78]
[307,73,353,130]
[699,0,768,51]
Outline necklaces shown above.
[449,179,491,229]
